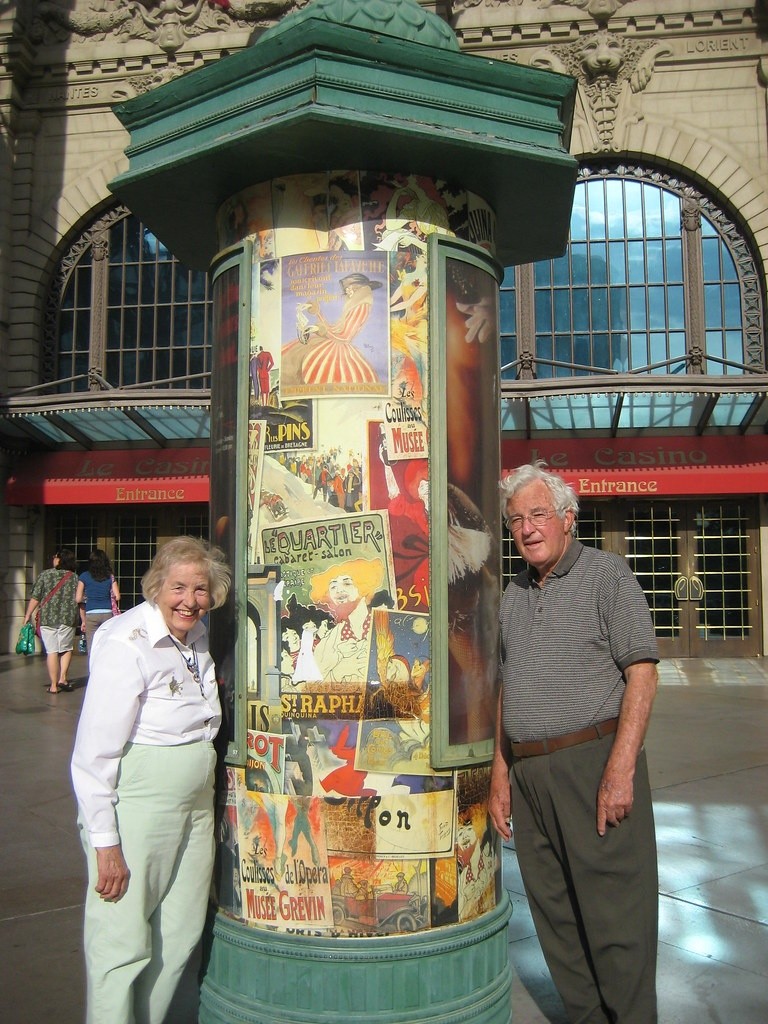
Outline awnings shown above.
[7,374,767,450]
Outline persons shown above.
[70,535,230,1024]
[486,460,659,1024]
[76,549,120,657]
[24,548,85,694]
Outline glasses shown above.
[505,508,565,530]
[52,556,60,559]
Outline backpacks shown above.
[34,610,42,638]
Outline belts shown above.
[511,717,618,757]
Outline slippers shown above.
[58,681,73,692]
[46,688,57,693]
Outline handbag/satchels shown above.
[78,631,87,652]
[110,574,121,616]
[16,622,35,656]
